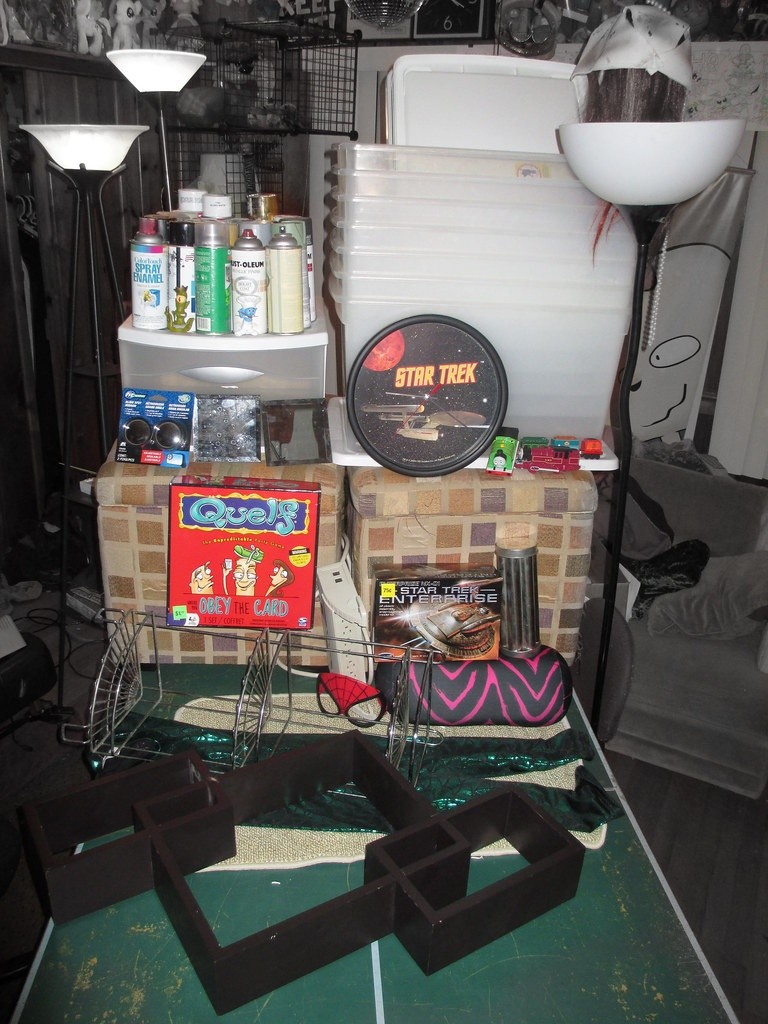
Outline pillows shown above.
[647,553,768,638]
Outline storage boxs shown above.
[329,138,637,443]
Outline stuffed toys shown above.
[75,0,203,55]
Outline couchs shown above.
[567,457,768,799]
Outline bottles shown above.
[129,188,318,336]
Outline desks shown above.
[96,443,351,667]
[345,464,599,668]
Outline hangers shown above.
[9,194,56,244]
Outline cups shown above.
[494,538,541,659]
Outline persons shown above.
[234,307,258,336]
